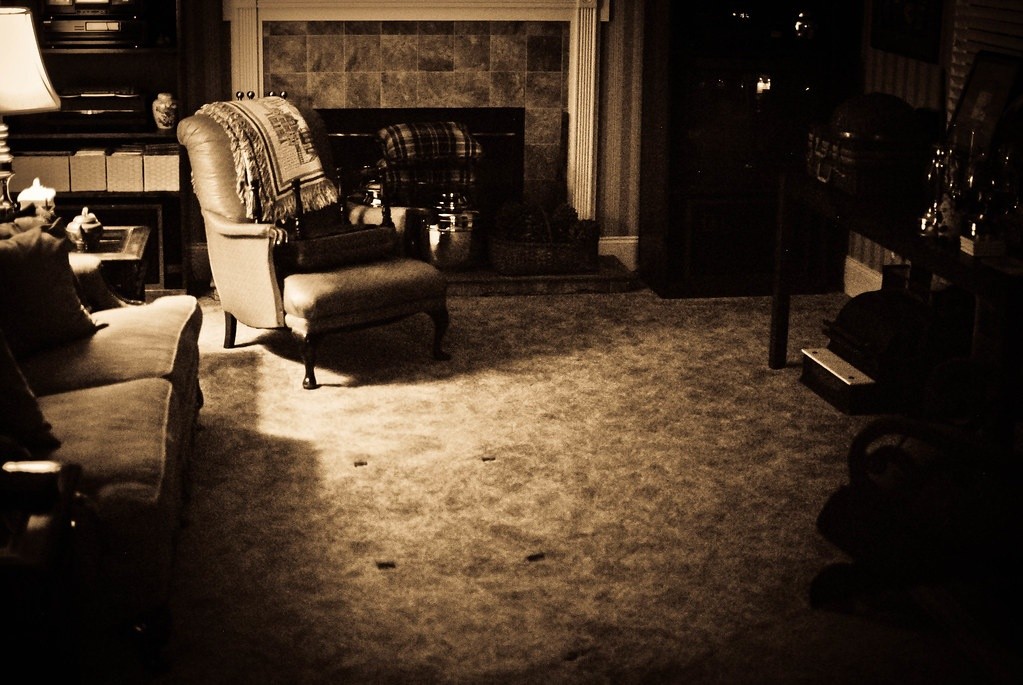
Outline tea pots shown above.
[66,207,103,251]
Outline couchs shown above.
[0,291,204,673]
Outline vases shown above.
[152,93,182,129]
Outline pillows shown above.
[0,216,110,452]
[376,121,483,160]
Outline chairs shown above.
[177,95,452,392]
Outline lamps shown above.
[0,6,62,217]
